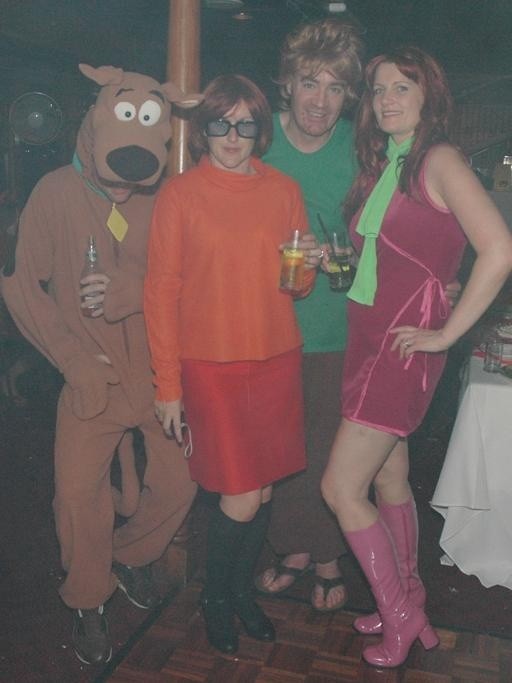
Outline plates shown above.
[480,343,512,358]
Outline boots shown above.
[200,500,276,655]
[340,498,440,668]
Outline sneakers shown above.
[72,606,114,667]
[113,563,164,611]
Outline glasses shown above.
[203,120,261,138]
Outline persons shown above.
[255,18,360,613]
[319,44,512,668]
[143,72,322,655]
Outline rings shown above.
[405,340,411,347]
[318,250,324,258]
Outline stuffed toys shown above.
[0,62,204,666]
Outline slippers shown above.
[254,559,349,613]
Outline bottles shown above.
[81,234,103,317]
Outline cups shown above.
[278,227,351,293]
[483,339,503,373]
